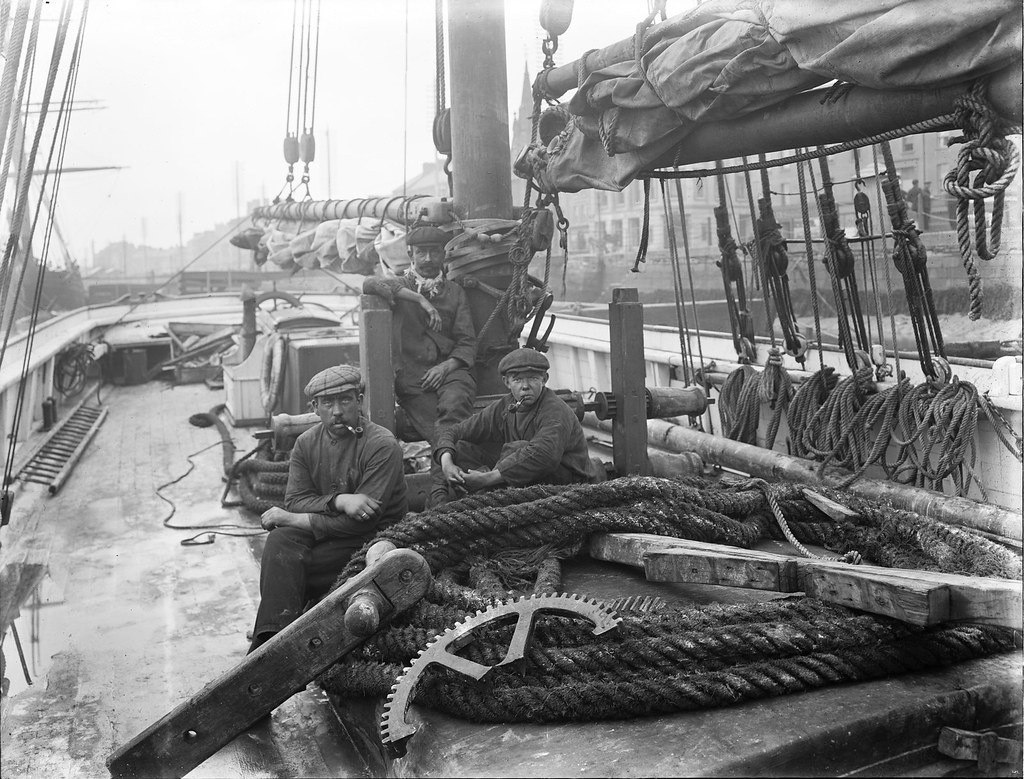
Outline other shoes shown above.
[246,635,270,654]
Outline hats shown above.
[498,348,549,375]
[304,365,360,398]
[406,226,448,247]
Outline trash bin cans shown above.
[123,348,148,385]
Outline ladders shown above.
[8,400,110,493]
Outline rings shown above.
[361,512,367,518]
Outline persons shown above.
[247,366,408,720]
[435,348,595,503]
[363,227,478,445]
